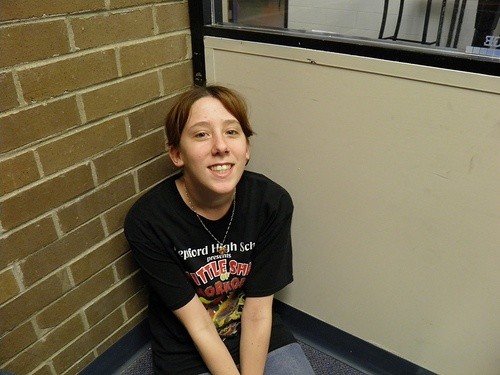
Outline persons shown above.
[123,82,318,375]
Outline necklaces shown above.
[182,181,237,255]
